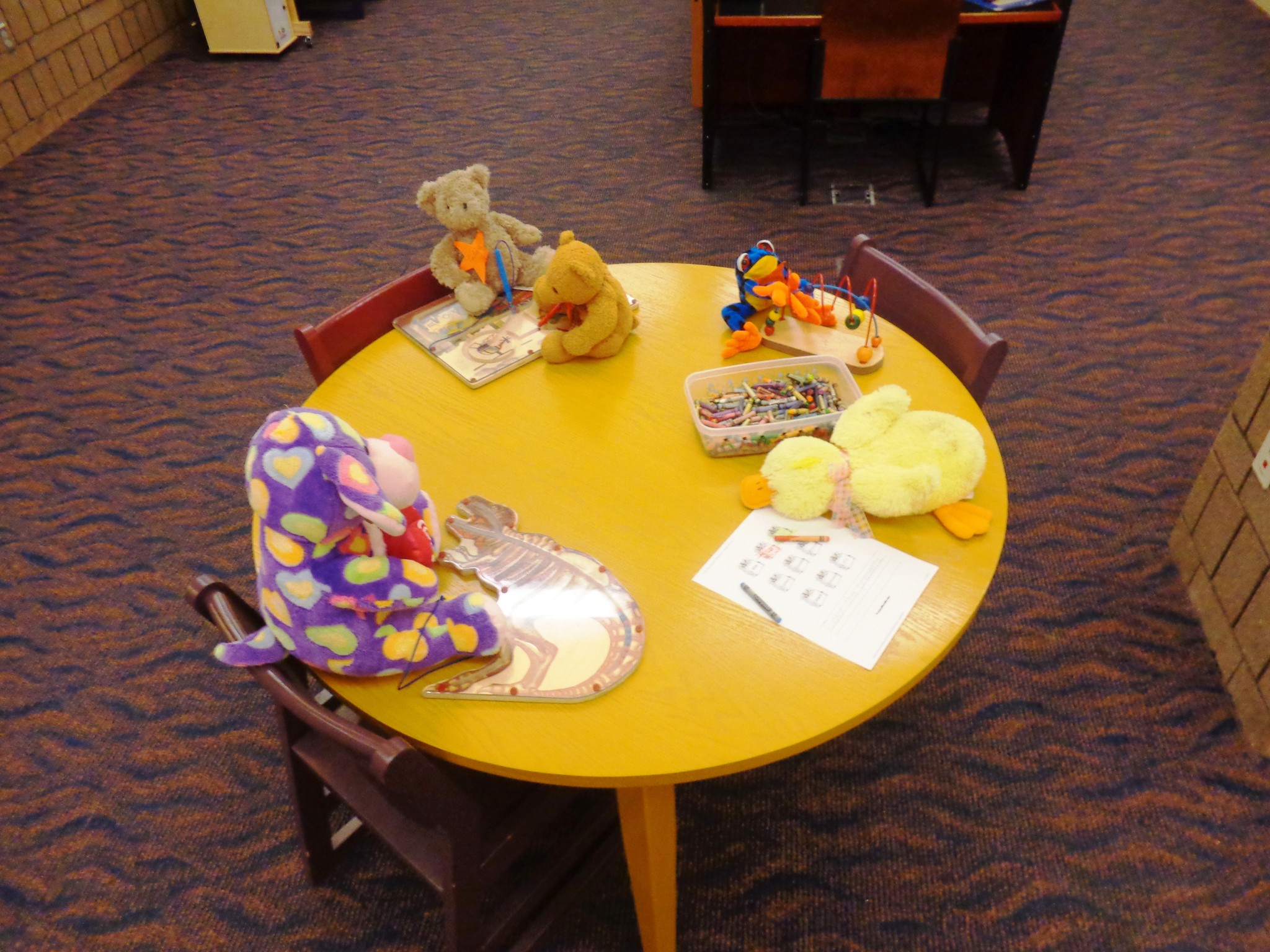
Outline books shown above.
[394,281,638,388]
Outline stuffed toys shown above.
[416,163,557,315]
[721,236,835,358]
[738,386,993,539]
[532,234,638,364]
[213,408,505,676]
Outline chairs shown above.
[292,261,454,389]
[184,572,621,951]
[834,232,1010,412]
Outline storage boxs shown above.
[684,354,861,457]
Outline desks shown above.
[251,261,1009,952]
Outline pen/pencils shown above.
[495,248,513,308]
